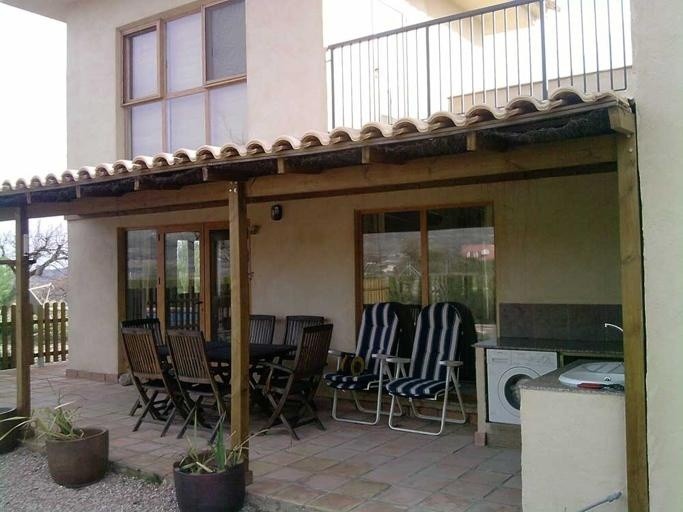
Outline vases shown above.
[0,406,18,454]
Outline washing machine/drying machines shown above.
[485,349,558,425]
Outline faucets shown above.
[604,322,623,332]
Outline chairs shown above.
[120,315,334,446]
[384,302,467,436]
[323,302,404,426]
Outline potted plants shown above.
[0,377,109,488]
[173,408,292,512]
